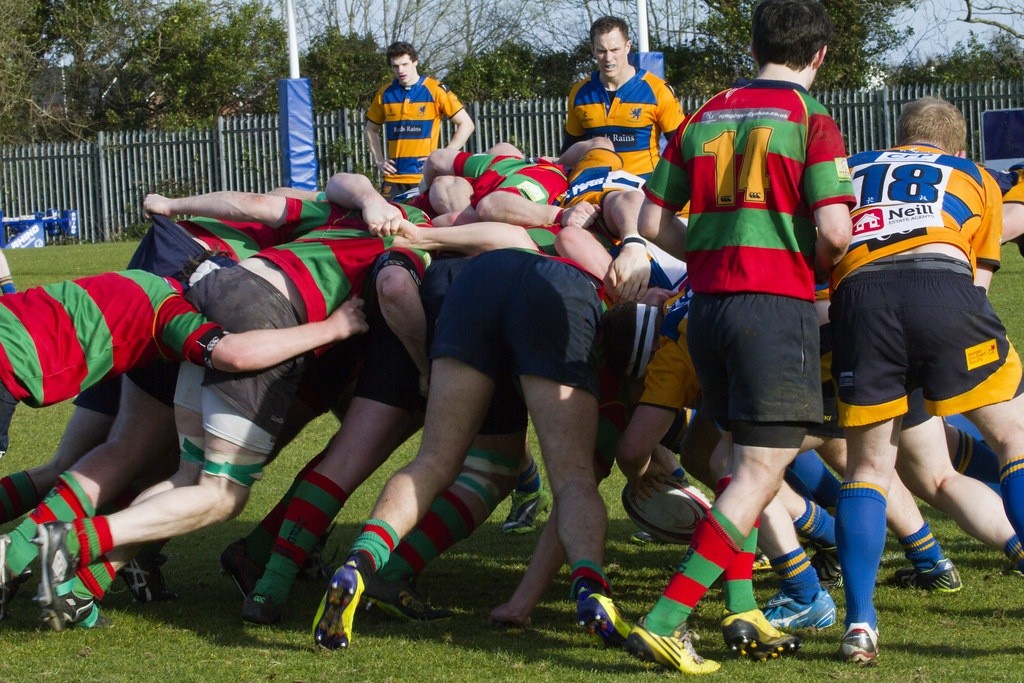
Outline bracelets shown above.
[553,207,570,228]
[619,237,647,248]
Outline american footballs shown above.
[620,475,715,546]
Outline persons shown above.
[826,96,1024,666]
[625,0,858,675]
[558,16,685,180]
[0,136,1024,648]
[363,42,475,203]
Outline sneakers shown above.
[838,621,879,662]
[219,537,265,601]
[31,520,80,632]
[575,583,635,648]
[753,552,772,572]
[630,530,663,545]
[798,535,843,587]
[359,572,456,623]
[501,478,550,535]
[50,577,115,629]
[242,591,286,629]
[720,607,804,661]
[624,614,721,676]
[895,558,963,593]
[309,550,372,654]
[761,586,838,629]
[119,552,179,603]
[0,533,32,632]
